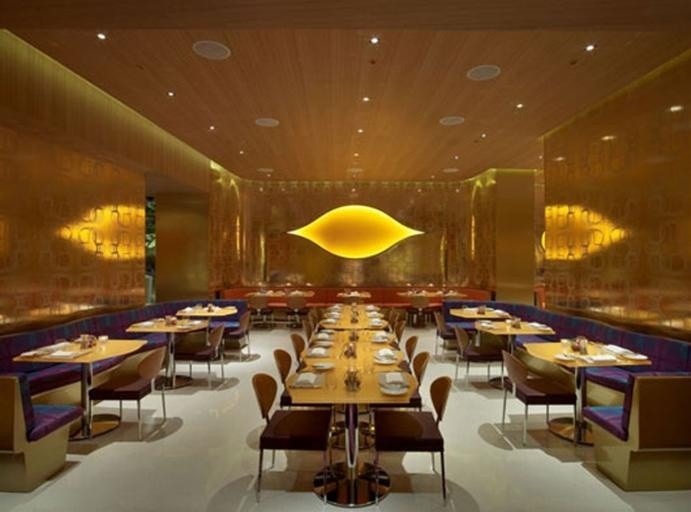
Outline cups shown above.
[99,335,109,353]
[511,317,522,328]
[81,334,95,348]
[571,336,590,355]
[343,367,364,389]
[479,303,486,313]
[343,301,361,359]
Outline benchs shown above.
[220,286,490,326]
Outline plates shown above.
[557,354,576,362]
[380,386,410,396]
[625,351,649,360]
[313,362,334,372]
[373,356,397,365]
[366,304,388,345]
[308,305,342,358]
